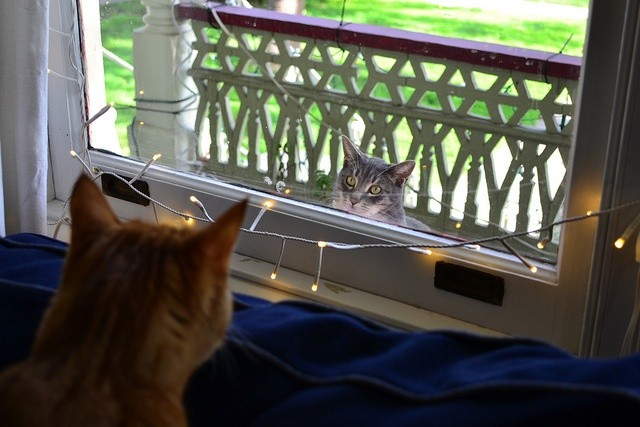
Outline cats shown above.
[0,170,250,427]
[324,134,442,237]
